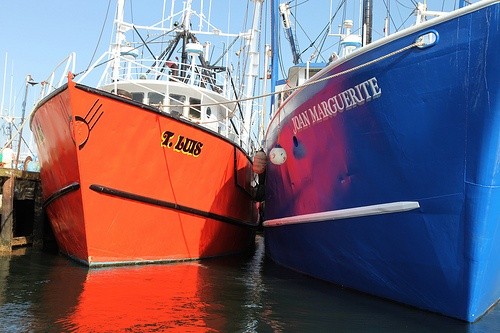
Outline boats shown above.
[1,0,500,321]
